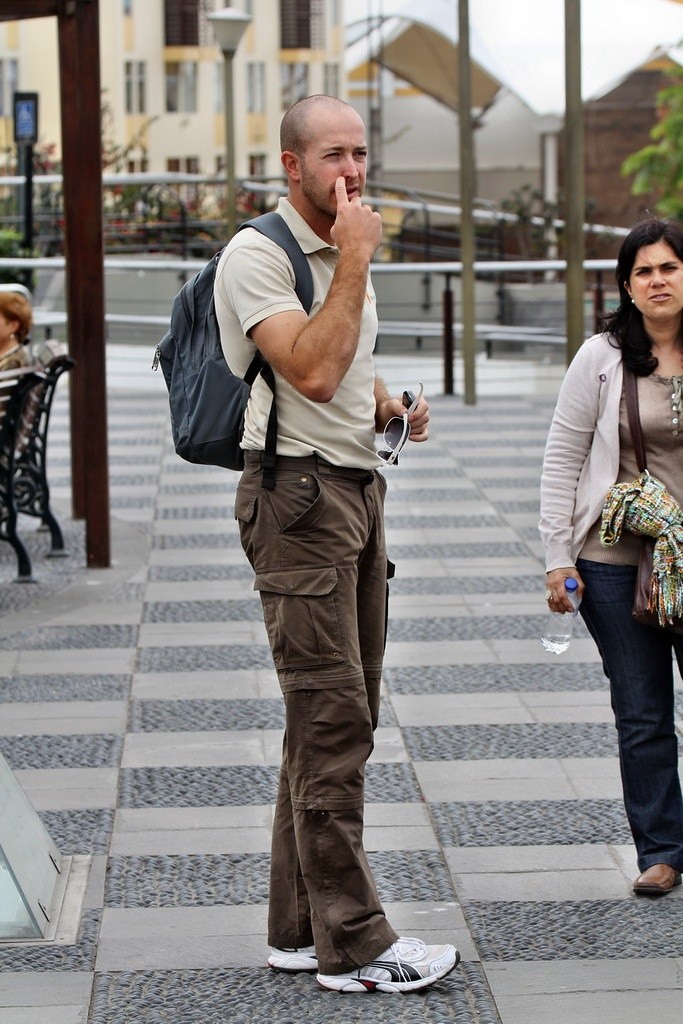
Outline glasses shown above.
[376,381,425,466]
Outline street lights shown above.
[206,6,253,247]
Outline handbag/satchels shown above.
[632,535,683,634]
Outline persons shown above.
[210,92,462,993]
[0,290,34,372]
[536,215,683,893]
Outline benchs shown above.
[0,354,77,584]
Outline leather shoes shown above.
[633,863,682,893]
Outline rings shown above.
[544,590,554,602]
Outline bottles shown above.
[539,577,580,655]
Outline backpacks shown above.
[158,211,316,468]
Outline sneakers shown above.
[267,946,319,973]
[316,937,462,992]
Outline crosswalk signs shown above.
[12,96,35,142]
